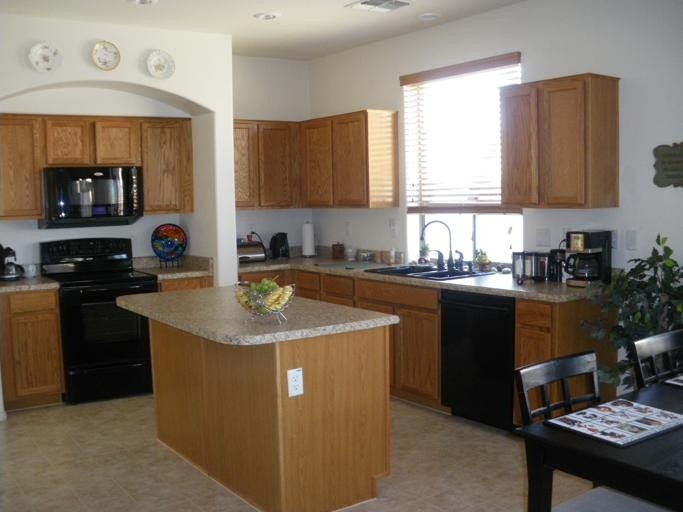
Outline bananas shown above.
[236,291,268,313]
[262,286,293,310]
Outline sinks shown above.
[374,265,435,277]
[420,269,476,278]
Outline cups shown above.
[357,251,369,264]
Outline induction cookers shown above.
[38,269,157,304]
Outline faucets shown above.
[419,220,455,268]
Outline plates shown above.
[150,224,188,259]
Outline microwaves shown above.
[37,164,144,230]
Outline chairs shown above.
[629,330,683,388]
[511,350,605,511]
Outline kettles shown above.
[268,231,289,260]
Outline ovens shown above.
[59,303,152,406]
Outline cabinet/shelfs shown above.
[239,269,293,289]
[298,108,397,209]
[44,116,140,166]
[1,288,64,410]
[140,117,193,214]
[514,299,555,425]
[0,114,44,220]
[498,73,619,208]
[233,120,298,210]
[354,279,440,400]
[293,271,354,304]
[159,275,214,293]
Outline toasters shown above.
[237,240,268,264]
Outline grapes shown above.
[250,279,279,293]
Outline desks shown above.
[512,378,683,511]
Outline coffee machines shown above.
[564,228,611,287]
[0,244,24,282]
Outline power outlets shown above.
[561,228,567,248]
[248,224,256,238]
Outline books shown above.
[666,373,683,387]
[547,397,683,452]
